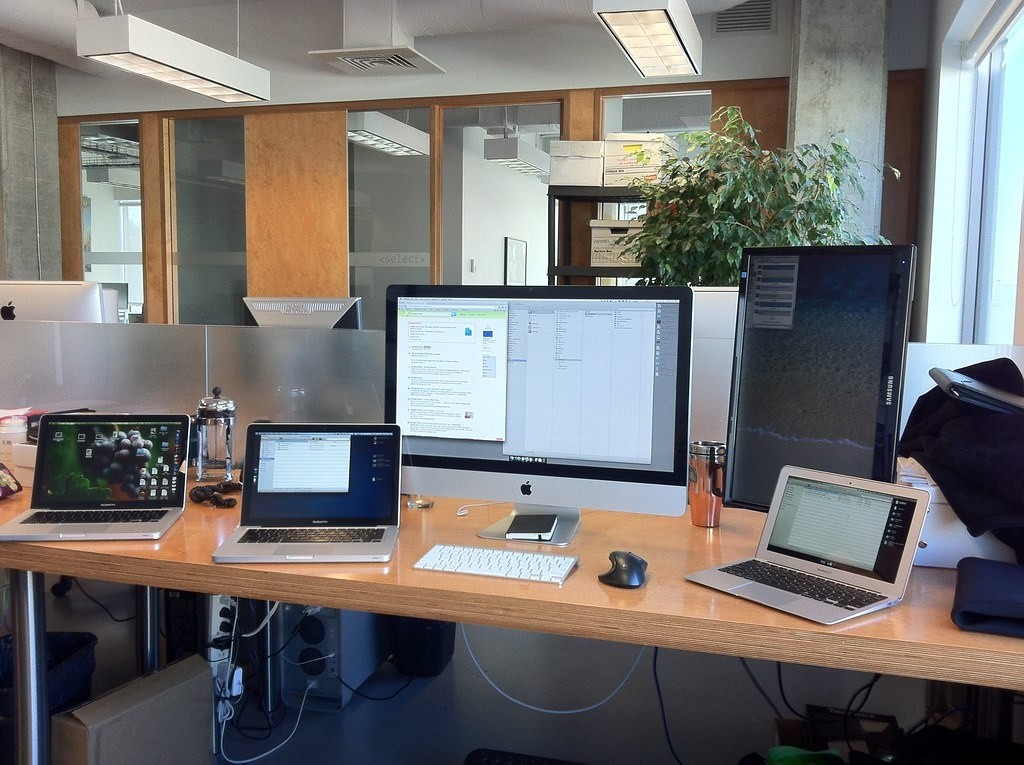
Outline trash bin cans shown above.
[0,623,99,765]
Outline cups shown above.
[690,441,728,527]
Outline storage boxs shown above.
[896,456,1018,570]
[849,740,869,756]
[772,718,905,765]
[827,740,850,764]
[549,141,605,185]
[52,653,214,765]
[589,219,648,268]
[804,705,899,730]
[605,132,679,157]
[602,156,673,187]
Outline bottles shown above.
[195,387,234,481]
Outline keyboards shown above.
[413,544,581,587]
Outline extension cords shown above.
[205,595,230,661]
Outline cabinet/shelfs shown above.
[547,185,665,287]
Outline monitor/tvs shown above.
[243,296,363,330]
[723,244,918,514]
[0,281,128,323]
[385,284,693,546]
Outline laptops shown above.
[0,412,190,543]
[211,422,401,563]
[684,466,930,624]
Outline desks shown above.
[0,435,1024,765]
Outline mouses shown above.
[599,551,647,589]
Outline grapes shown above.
[86,429,152,497]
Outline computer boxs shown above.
[278,601,378,713]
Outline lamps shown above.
[592,0,703,78]
[75,14,269,103]
[347,111,428,157]
[484,129,549,176]
